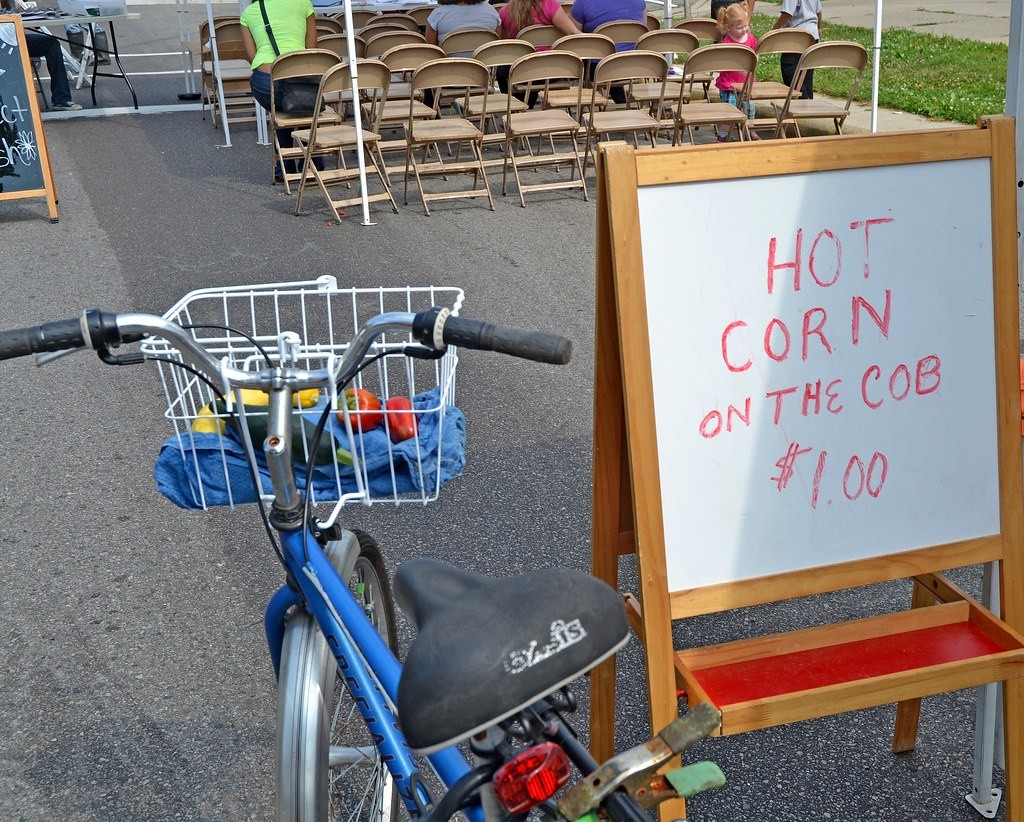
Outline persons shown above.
[0,0,83,111]
[716,1,762,144]
[567,0,648,104]
[238,0,326,185]
[770,0,822,99]
[498,0,583,109]
[424,0,501,110]
[710,0,756,44]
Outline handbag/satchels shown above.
[279,75,326,116]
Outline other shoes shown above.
[716,133,736,143]
[275,177,287,182]
[304,177,316,182]
[744,131,761,140]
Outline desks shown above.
[23,12,141,110]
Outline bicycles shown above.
[0,273,731,822]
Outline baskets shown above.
[139,275,465,506]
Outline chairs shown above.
[201,0,870,226]
[30,57,49,111]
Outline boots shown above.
[88,30,111,66]
[66,30,83,62]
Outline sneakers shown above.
[52,100,82,110]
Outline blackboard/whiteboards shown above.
[587,111,1024,623]
[0,14,56,200]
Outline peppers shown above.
[336,389,383,433]
[383,396,417,443]
